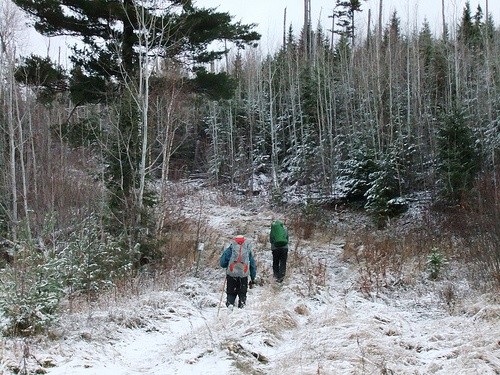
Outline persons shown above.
[270,220,289,283]
[220,235,257,310]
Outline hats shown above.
[276,220,283,226]
[236,234,244,238]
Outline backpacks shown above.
[226,238,250,278]
[271,223,288,247]
